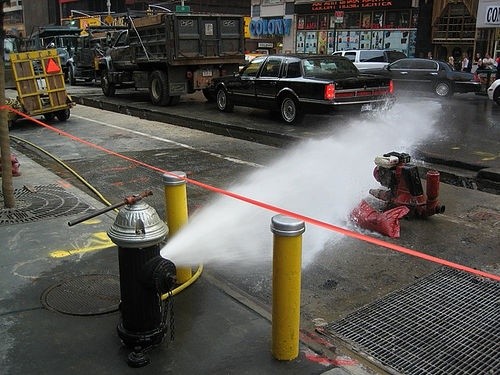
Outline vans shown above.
[331,49,407,70]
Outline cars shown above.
[203,55,393,125]
[360,58,480,97]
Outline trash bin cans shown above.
[477,63,497,96]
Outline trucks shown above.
[98,14,244,106]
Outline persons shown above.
[447,56,455,71]
[482,53,498,84]
[428,51,433,59]
[461,52,469,72]
[473,52,482,69]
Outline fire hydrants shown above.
[107,195,177,367]
